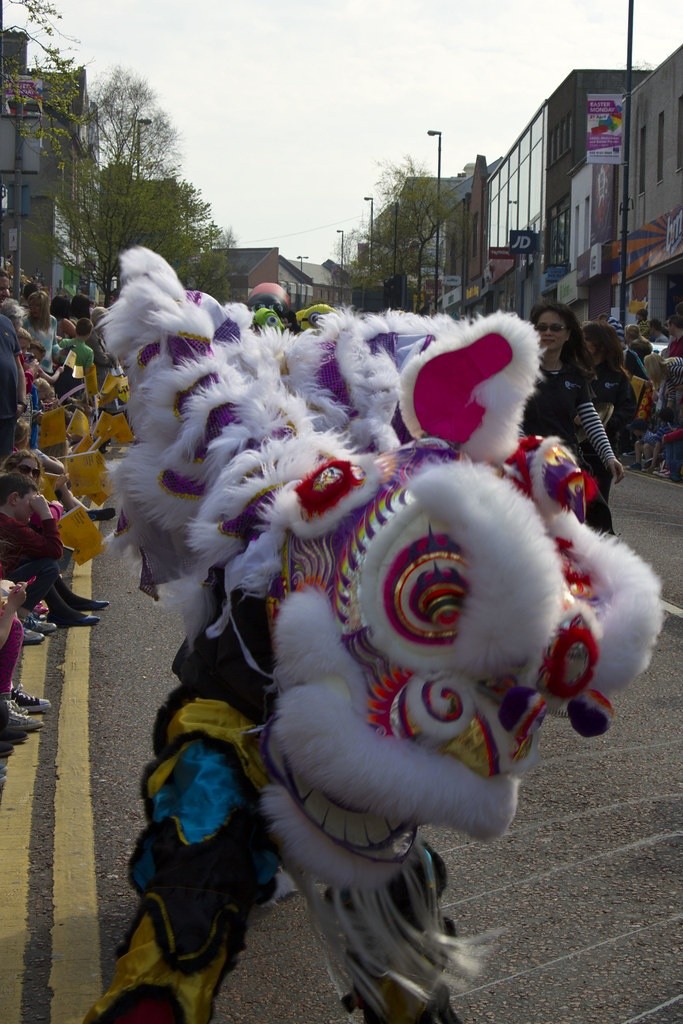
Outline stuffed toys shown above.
[89,246,665,1024]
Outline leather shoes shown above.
[46,609,101,625]
[72,600,109,610]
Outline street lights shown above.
[297,256,309,309]
[137,119,153,174]
[427,130,442,314]
[337,230,343,304]
[364,197,374,276]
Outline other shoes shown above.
[622,451,670,478]
[87,508,116,521]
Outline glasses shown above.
[18,464,40,478]
[536,323,568,334]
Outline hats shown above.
[626,419,645,430]
[660,407,674,422]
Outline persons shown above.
[597,303,683,482]
[0,259,120,784]
[519,303,637,535]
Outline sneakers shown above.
[0,687,52,757]
[23,628,45,645]
[22,616,56,633]
